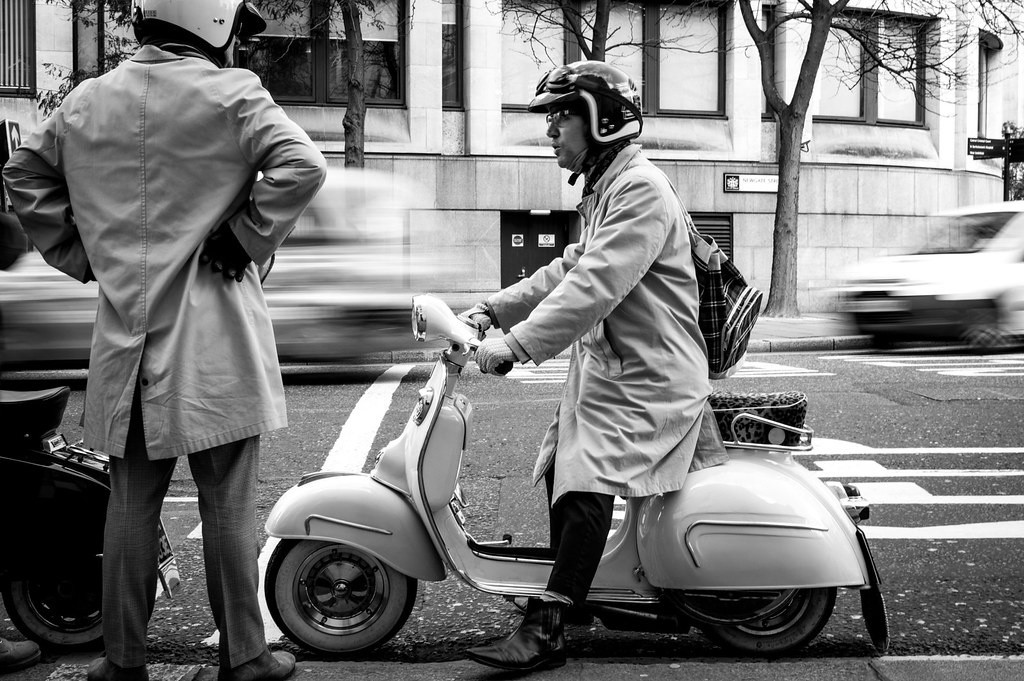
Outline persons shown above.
[3,0,328,681]
[459,60,711,673]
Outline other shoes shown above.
[218,651,295,681]
[0,636,41,674]
[87,656,150,681]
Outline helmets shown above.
[529,61,643,143]
[130,0,267,53]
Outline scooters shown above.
[0,383,183,655]
[265,291,892,660]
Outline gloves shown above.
[474,337,519,376]
[199,222,252,282]
[460,303,491,330]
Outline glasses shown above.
[535,66,643,135]
[546,108,585,127]
[235,22,243,36]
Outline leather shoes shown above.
[464,598,567,671]
[513,597,594,626]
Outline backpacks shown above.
[626,164,763,380]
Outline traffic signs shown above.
[968,137,1024,164]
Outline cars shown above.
[833,201,1024,354]
